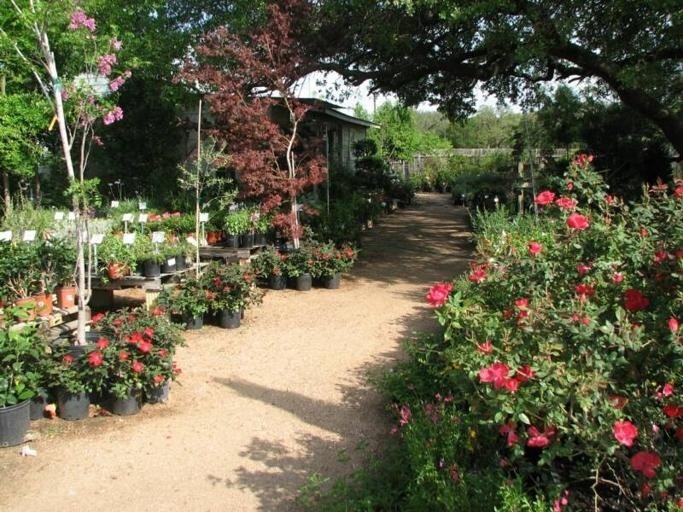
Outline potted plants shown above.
[171,279,205,330]
[30,336,50,420]
[98,210,289,279]
[0,325,36,447]
[288,247,313,290]
[0,238,76,322]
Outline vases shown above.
[267,268,287,289]
[57,389,90,421]
[107,386,142,415]
[219,308,241,328]
[144,379,172,405]
[323,270,341,289]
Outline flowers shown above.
[87,307,188,388]
[314,245,353,264]
[205,265,269,317]
[52,349,83,387]
